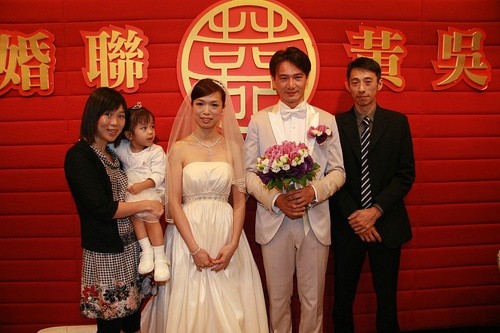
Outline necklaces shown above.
[191,131,221,157]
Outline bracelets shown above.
[189,247,202,256]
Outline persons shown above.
[329,57,416,333]
[140,78,270,333]
[78,103,171,282]
[245,46,346,333]
[64,87,164,333]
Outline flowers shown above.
[252,139,321,216]
[306,124,336,146]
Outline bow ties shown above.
[279,102,308,121]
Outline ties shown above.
[359,116,374,210]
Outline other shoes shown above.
[137,247,155,275]
[153,254,172,282]
